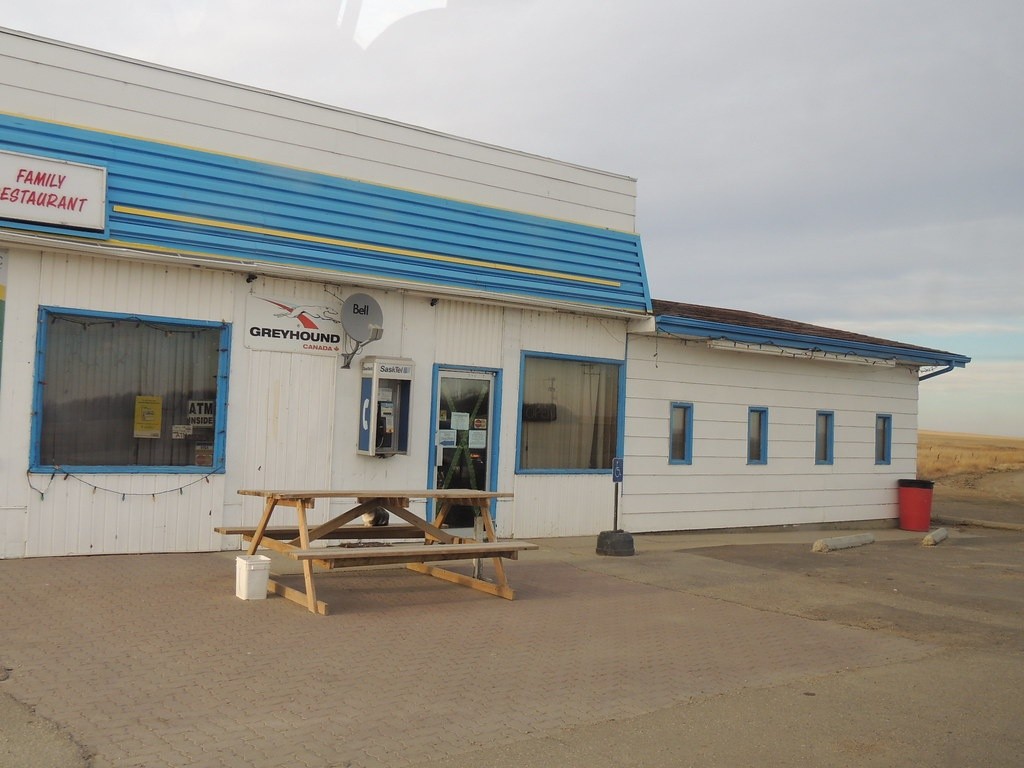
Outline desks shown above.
[237,487,517,615]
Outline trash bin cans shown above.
[898,478,935,532]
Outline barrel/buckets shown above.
[234,554,272,601]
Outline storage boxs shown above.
[189,440,213,466]
[232,553,271,603]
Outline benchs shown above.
[214,521,454,535]
[283,539,539,562]
